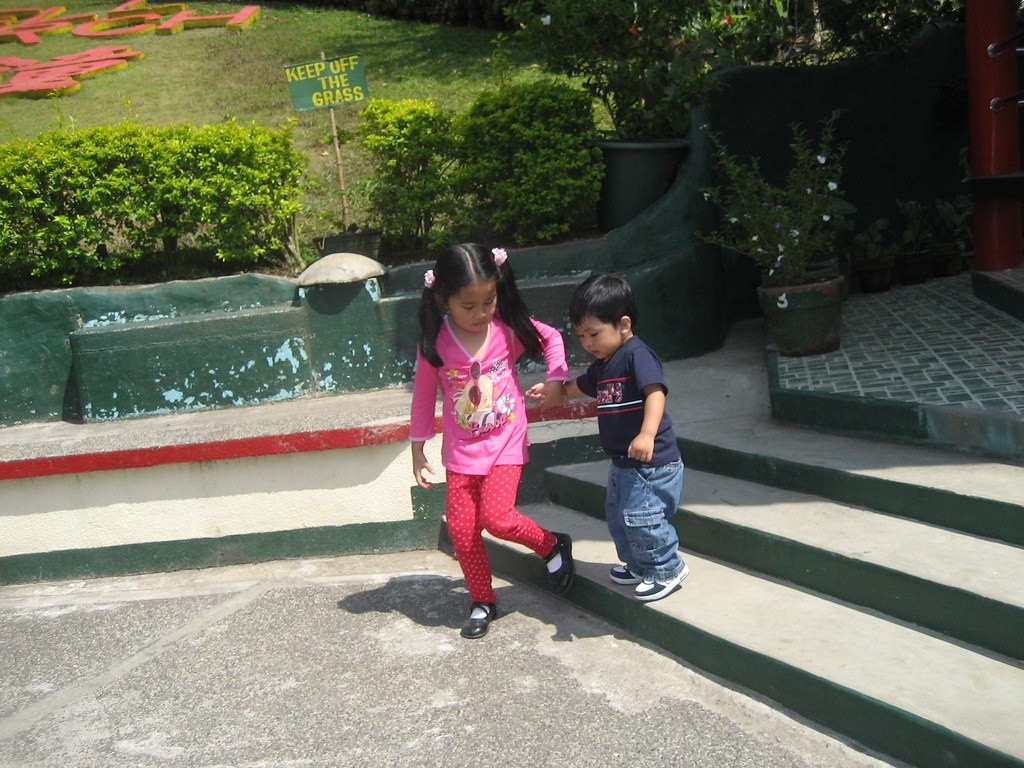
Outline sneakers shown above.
[609,564,643,585]
[633,563,690,602]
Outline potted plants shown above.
[502,0,695,237]
[826,194,972,298]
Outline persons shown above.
[408,241,576,640]
[523,272,691,602]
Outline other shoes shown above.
[544,532,575,596]
[461,602,496,639]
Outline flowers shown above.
[689,108,843,288]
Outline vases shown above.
[313,228,382,260]
[756,271,842,356]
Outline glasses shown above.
[468,359,482,407]
